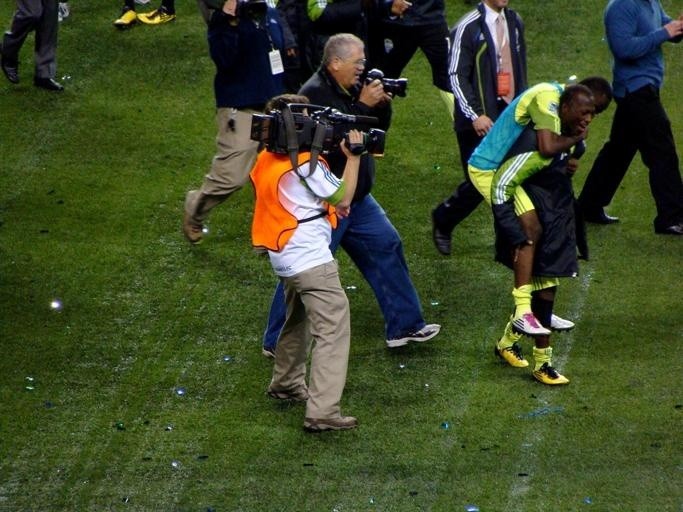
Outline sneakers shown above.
[2,56,22,84]
[652,218,683,237]
[430,209,455,258]
[383,323,441,351]
[33,75,64,93]
[182,189,208,249]
[575,197,622,226]
[300,412,360,433]
[493,310,577,390]
[259,344,275,362]
[112,6,177,30]
[263,383,311,402]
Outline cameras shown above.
[365,68,407,98]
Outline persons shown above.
[110,2,177,32]
[430,2,528,252]
[490,85,596,388]
[181,1,300,248]
[262,33,443,360]
[246,93,358,432]
[0,0,65,92]
[465,75,613,337]
[57,2,71,22]
[198,1,455,120]
[575,1,682,235]
